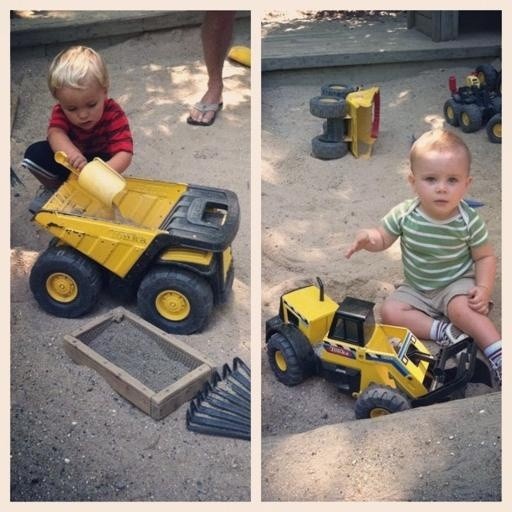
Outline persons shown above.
[265,277,473,419]
[187,11,237,126]
[444,64,502,143]
[54,150,126,208]
[21,46,134,214]
[28,170,239,335]
[309,82,380,161]
[345,129,502,386]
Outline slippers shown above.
[186,100,223,127]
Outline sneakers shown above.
[29,183,57,213]
[487,358,502,390]
[436,321,472,369]
